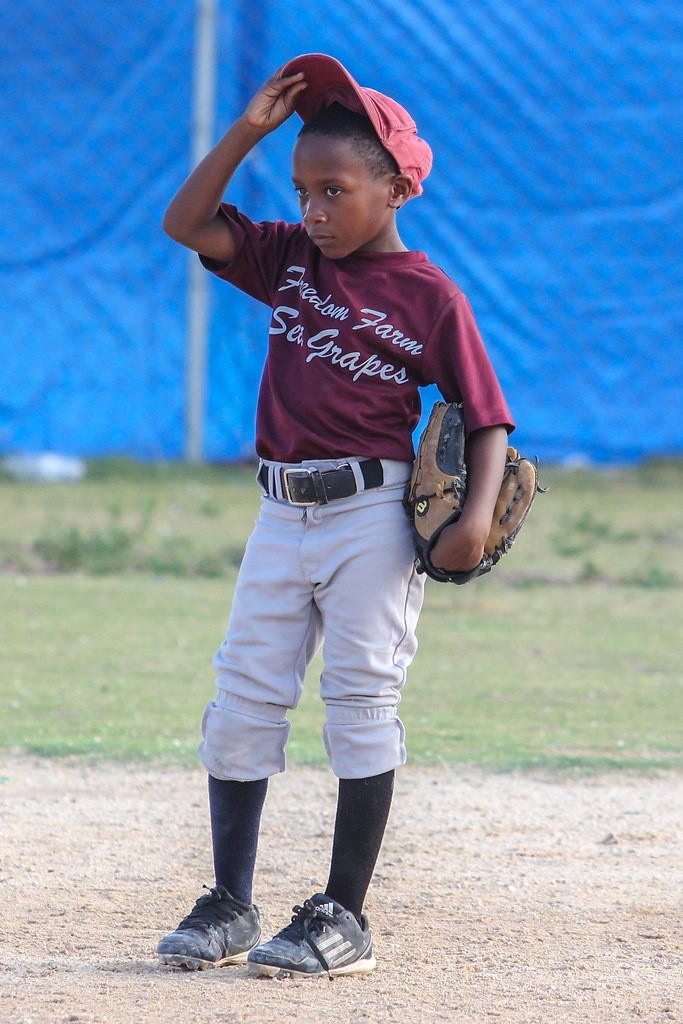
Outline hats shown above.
[280,53,433,210]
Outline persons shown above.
[154,52,516,977]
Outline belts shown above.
[259,458,383,507]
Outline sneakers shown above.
[157,884,261,972]
[246,893,376,981]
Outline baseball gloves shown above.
[407,397,541,583]
